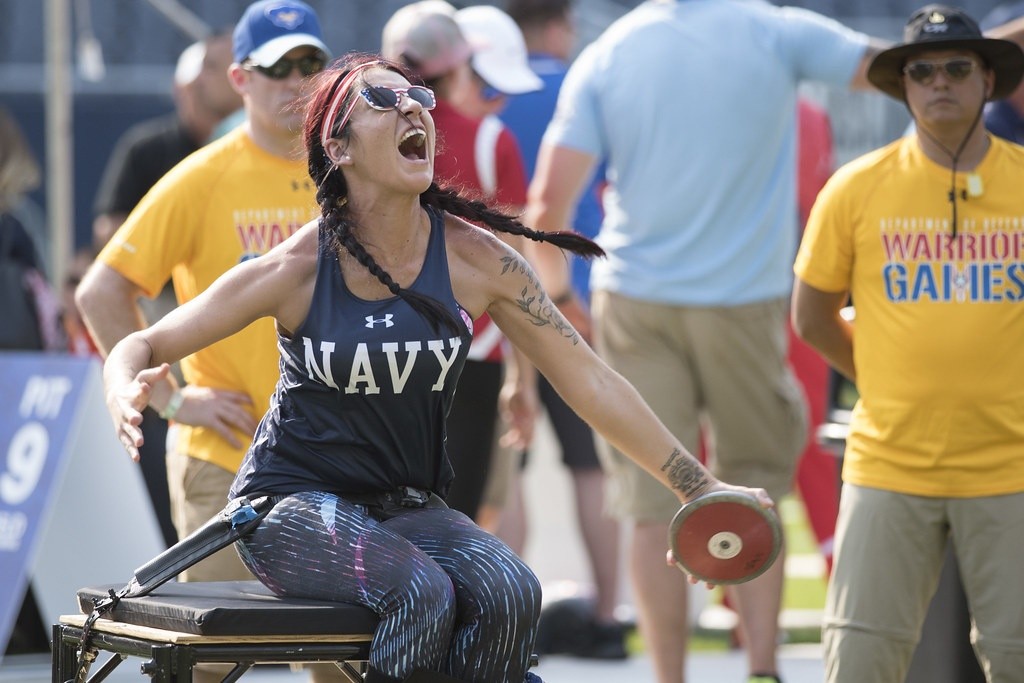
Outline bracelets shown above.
[157,388,185,419]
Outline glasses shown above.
[242,54,324,79]
[902,59,984,86]
[334,86,437,136]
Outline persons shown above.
[101,49,775,682]
[73,0,1024,683]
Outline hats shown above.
[229,0,331,69]
[867,3,1024,105]
[456,5,545,94]
[381,0,475,79]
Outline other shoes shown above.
[539,596,637,661]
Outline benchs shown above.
[52,579,539,683]
